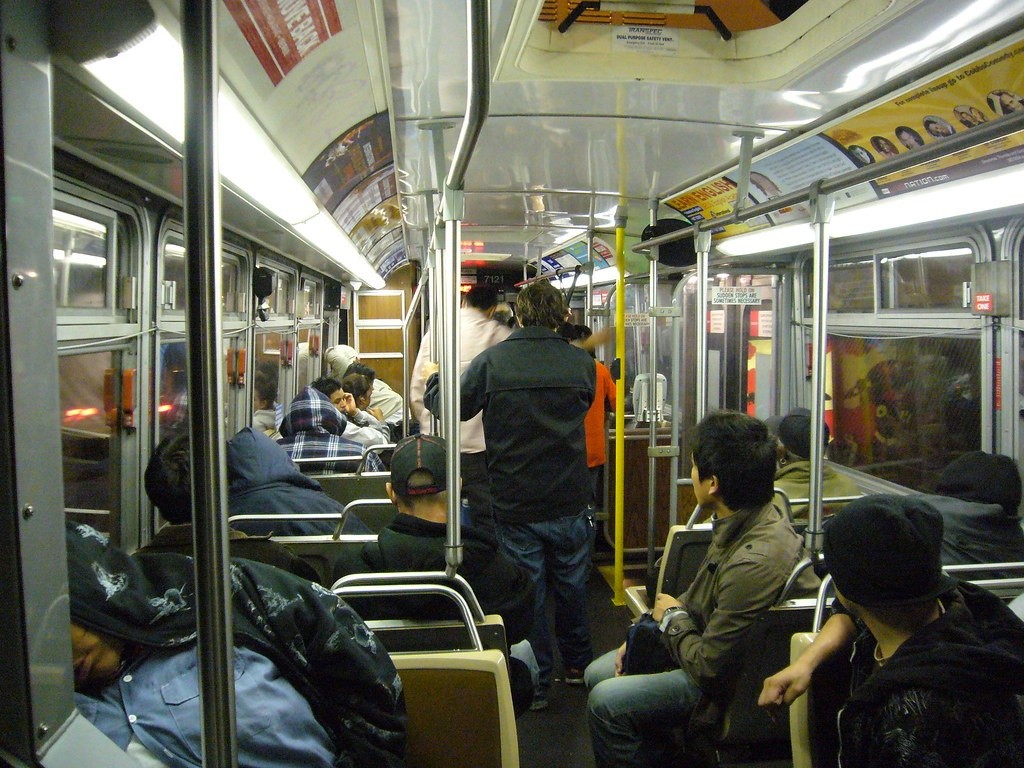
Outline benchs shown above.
[602,411,1024,768]
[137,369,521,766]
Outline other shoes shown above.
[555,662,585,683]
[529,699,549,711]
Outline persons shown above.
[758,494,1024,768]
[66,521,408,768]
[770,408,863,547]
[145,283,616,712]
[907,451,1024,580]
[584,407,825,768]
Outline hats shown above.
[762,408,831,458]
[824,492,959,605]
[938,451,1022,522]
[390,435,447,497]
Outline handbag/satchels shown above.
[623,608,682,675]
